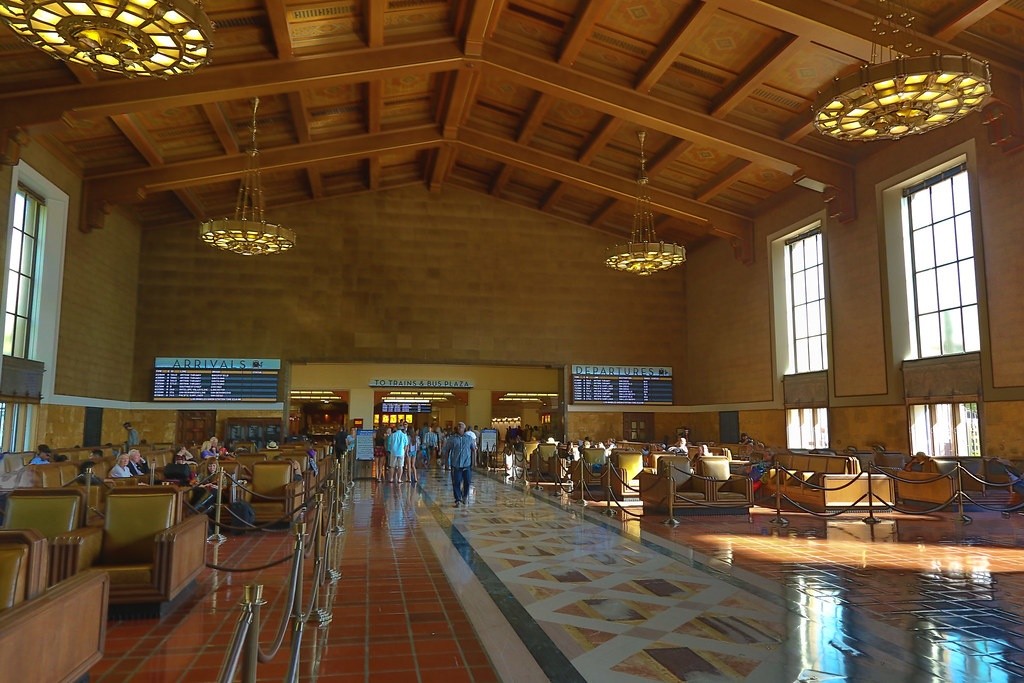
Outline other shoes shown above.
[463,499,468,504]
[456,502,461,507]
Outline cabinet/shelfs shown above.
[222,417,285,445]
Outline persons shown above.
[27,419,926,537]
[440,422,477,509]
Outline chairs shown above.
[521,441,959,516]
[0,439,330,683]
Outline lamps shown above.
[600,130,687,277]
[810,0,993,143]
[197,97,297,256]
[0,0,216,80]
